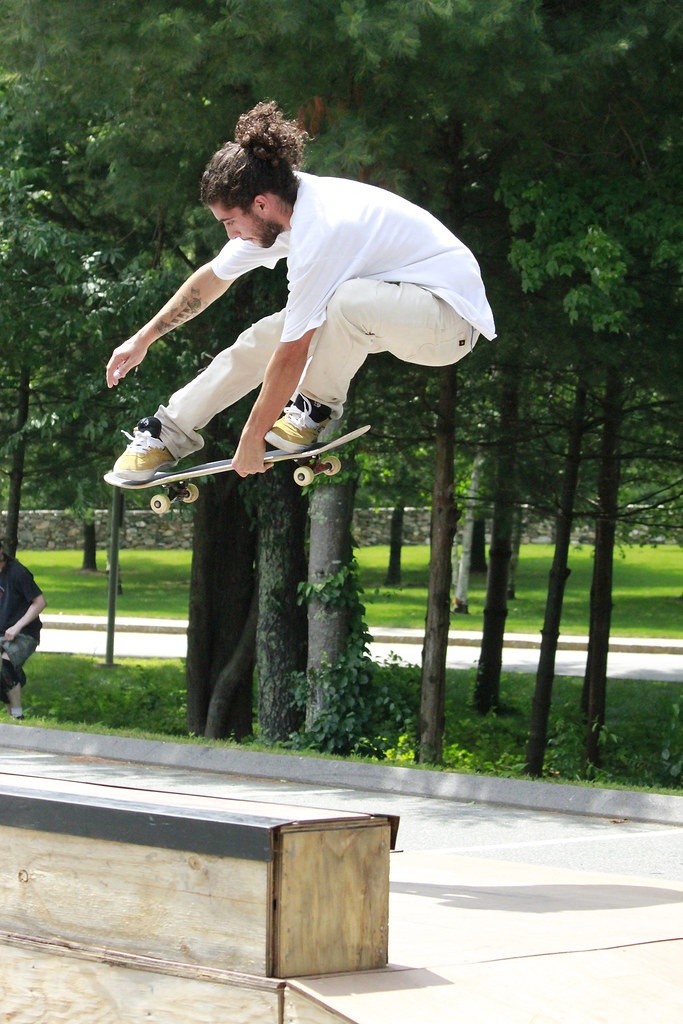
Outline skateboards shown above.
[103,425,371,514]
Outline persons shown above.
[0,546,46,719]
[106,102,497,481]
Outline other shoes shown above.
[11,708,24,721]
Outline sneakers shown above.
[263,391,333,454]
[114,416,180,481]
